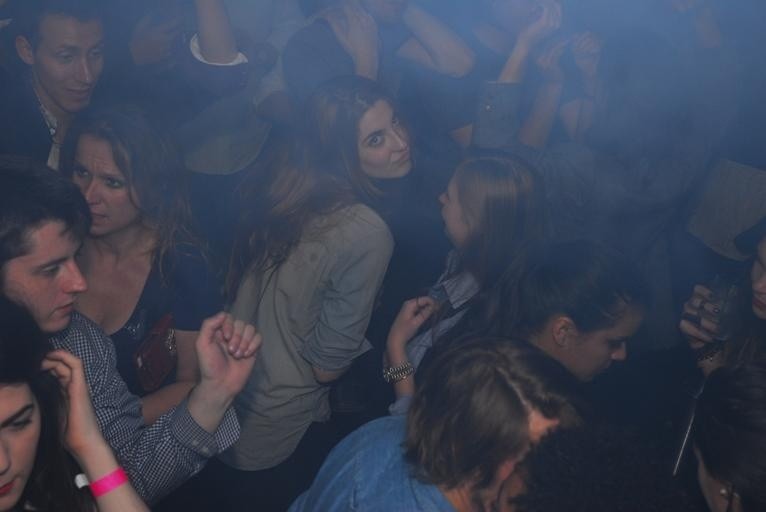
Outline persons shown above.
[0,2,765,512]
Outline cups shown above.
[701,275,742,343]
